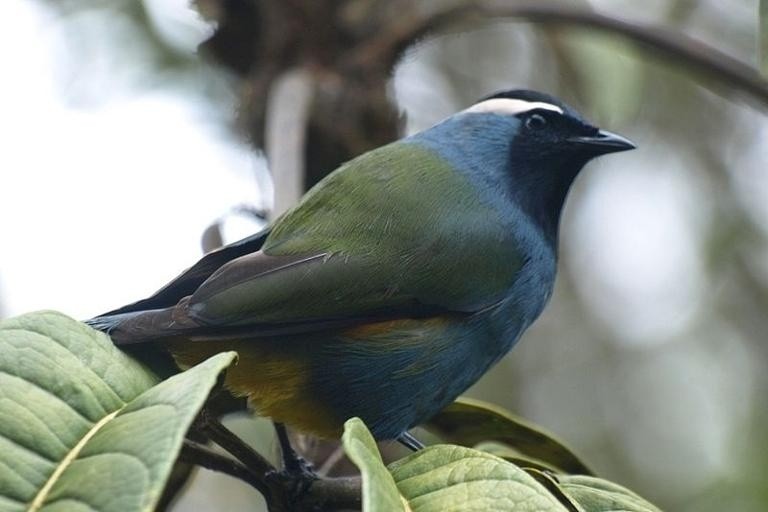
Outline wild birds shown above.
[81,88,638,503]
